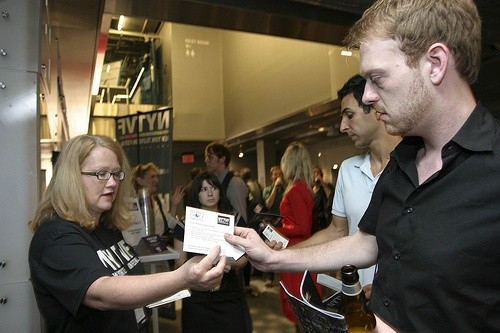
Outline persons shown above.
[224,0,500,333]
[240,166,333,231]
[262,141,323,321]
[189,142,249,289]
[263,74,409,304]
[172,172,253,333]
[131,163,185,246]
[28,134,226,333]
[182,166,203,214]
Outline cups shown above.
[137,188,154,237]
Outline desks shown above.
[140,246,179,333]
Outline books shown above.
[278,270,348,332]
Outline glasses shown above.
[81,170,125,181]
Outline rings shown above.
[211,288,213,292]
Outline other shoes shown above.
[264,280,272,286]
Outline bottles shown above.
[336,264,378,333]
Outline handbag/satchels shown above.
[159,227,175,268]
